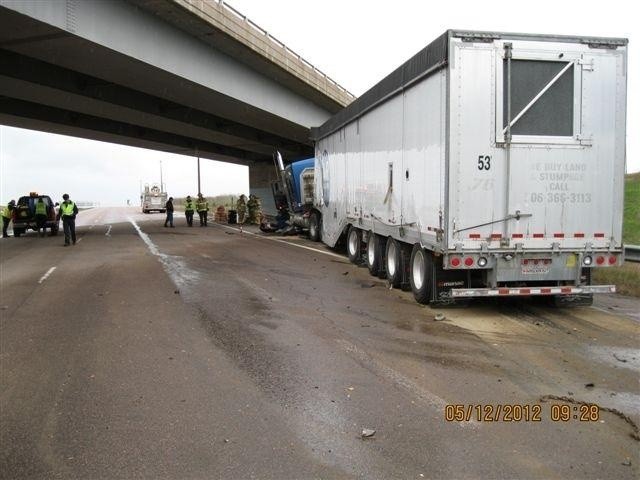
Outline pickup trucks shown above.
[12,192,59,236]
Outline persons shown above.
[164,197,176,228]
[260,203,312,236]
[1,194,78,246]
[185,193,209,227]
[237,193,259,225]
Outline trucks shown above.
[270,28,629,308]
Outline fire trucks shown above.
[141,183,169,214]
